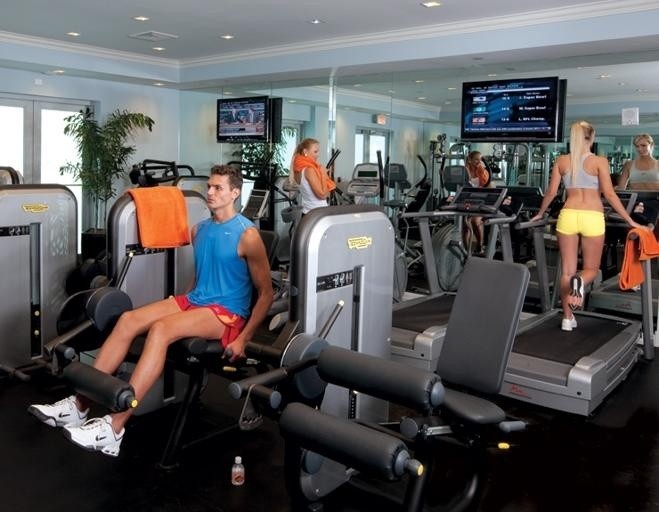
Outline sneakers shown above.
[62,415,125,456]
[562,313,577,331]
[27,396,90,428]
[567,275,584,311]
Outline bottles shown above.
[504,196,512,205]
[633,202,647,214]
[230,457,246,484]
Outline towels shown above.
[294,154,338,193]
[619,226,659,290]
[478,162,490,185]
[124,186,192,249]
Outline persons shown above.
[288,136,337,218]
[243,105,261,126]
[26,164,275,460]
[224,107,241,125]
[462,148,492,257]
[529,120,655,334]
[613,130,659,192]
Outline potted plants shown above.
[59,104,156,263]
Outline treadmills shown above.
[501,187,653,416]
[514,188,658,320]
[391,188,537,373]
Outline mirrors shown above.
[182,52,659,326]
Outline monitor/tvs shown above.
[216,96,269,142]
[449,187,508,212]
[614,190,659,222]
[557,79,567,142]
[461,76,559,142]
[269,98,282,143]
[600,192,639,221]
[496,186,544,210]
